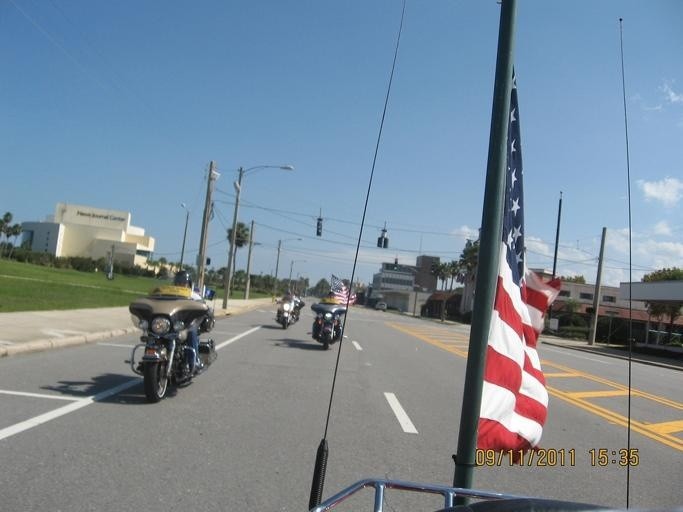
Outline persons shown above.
[173,269,206,375]
[311,289,344,341]
[293,290,302,307]
[274,289,296,321]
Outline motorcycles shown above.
[125,285,217,403]
[310,298,347,348]
[273,292,306,328]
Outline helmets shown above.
[174,271,192,288]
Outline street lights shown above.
[218,158,299,311]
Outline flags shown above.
[330,273,357,306]
[475,64,561,463]
[203,287,215,302]
